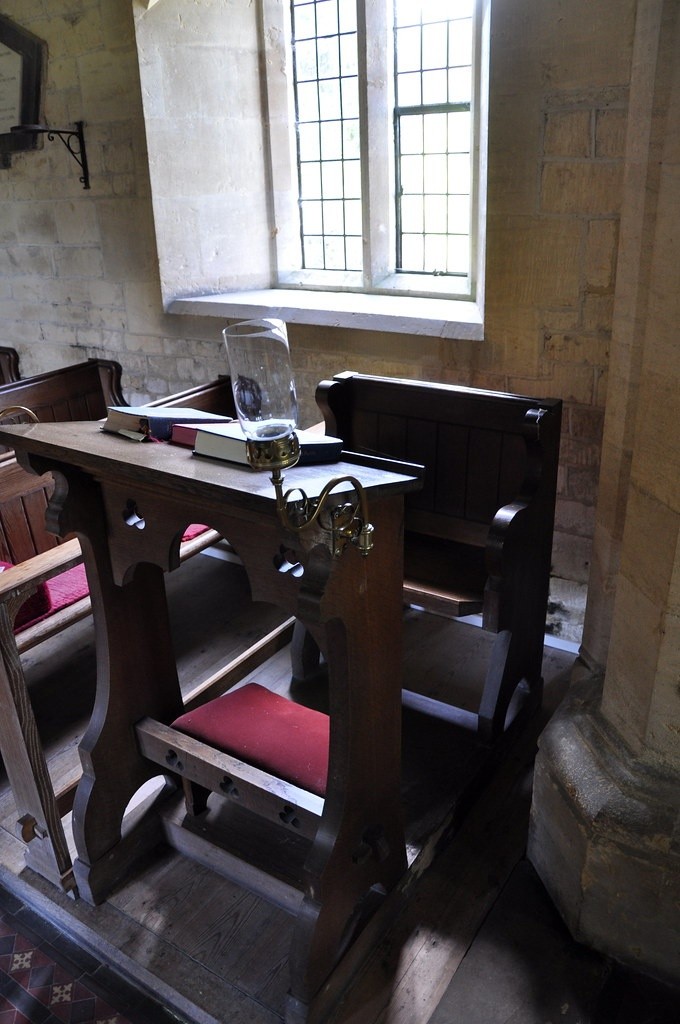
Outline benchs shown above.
[309,369,563,763]
[0,345,263,574]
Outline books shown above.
[100,405,342,470]
